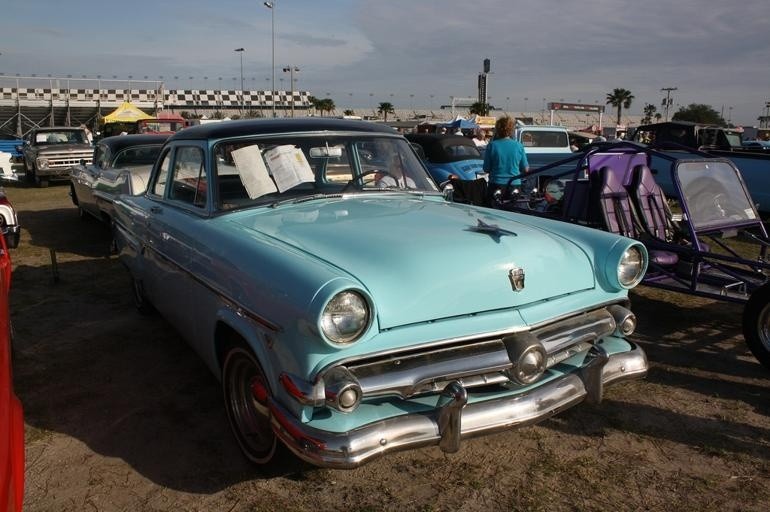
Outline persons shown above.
[484,117,530,210]
[81,123,138,145]
[742,132,770,150]
[377,154,416,189]
[570,130,657,155]
[395,125,488,148]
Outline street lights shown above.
[235,49,244,120]
[282,64,299,117]
[263,2,275,117]
[662,88,676,123]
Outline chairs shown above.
[596,164,709,267]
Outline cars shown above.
[401,133,490,184]
[111,116,650,469]
[0,116,239,253]
[512,120,769,217]
